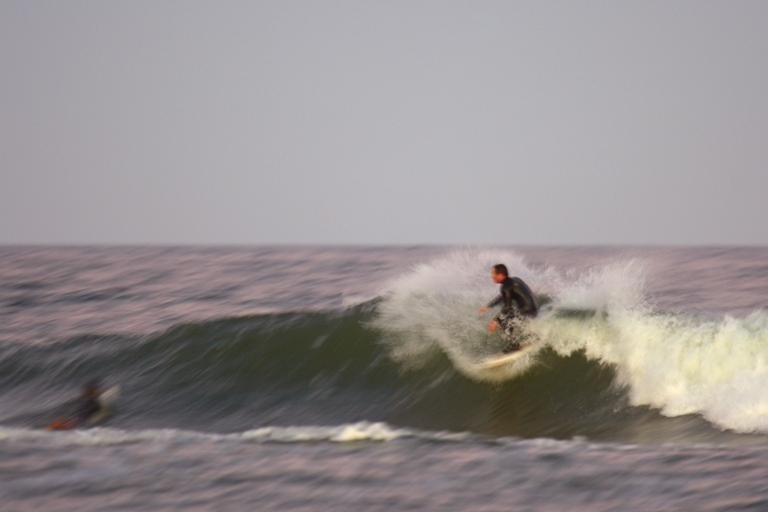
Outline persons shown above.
[51,381,103,430]
[478,263,538,354]
